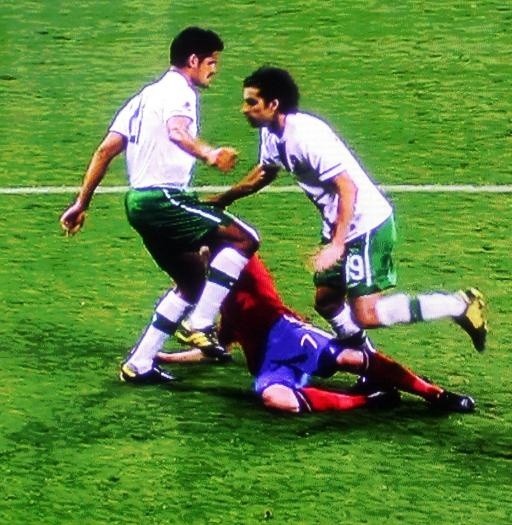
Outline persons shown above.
[58,26,260,383]
[155,245,476,416]
[205,62,487,393]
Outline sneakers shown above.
[452,286,486,352]
[436,387,475,411]
[174,320,233,361]
[119,363,176,384]
[346,376,401,409]
